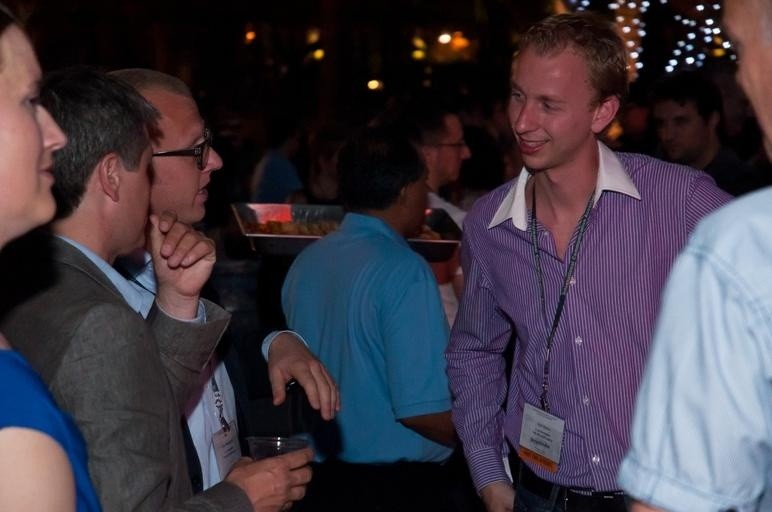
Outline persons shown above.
[282,128,470,511]
[615,1,772,512]
[254,115,348,204]
[652,67,767,198]
[0,65,312,512]
[445,12,734,512]
[0,5,102,512]
[102,68,339,492]
[389,89,472,329]
[455,87,525,192]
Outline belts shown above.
[512,459,625,511]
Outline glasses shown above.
[432,141,467,151]
[151,127,212,170]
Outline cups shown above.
[245,435,309,461]
